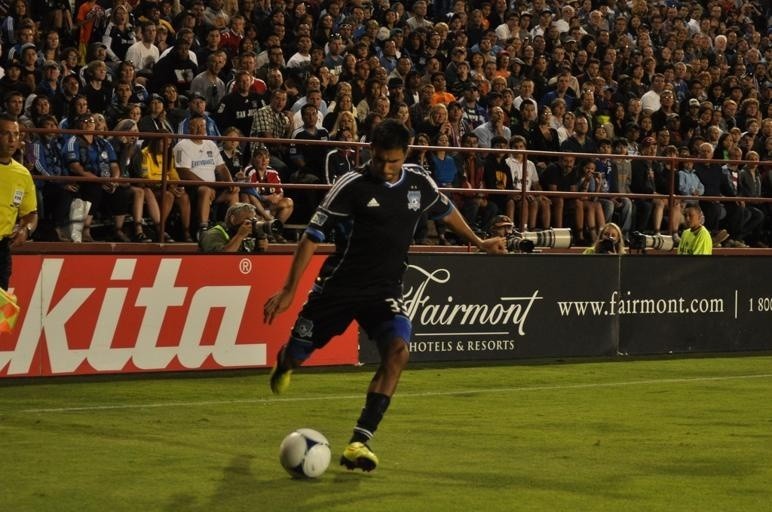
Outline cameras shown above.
[248,216,283,237]
[508,227,574,247]
[631,232,675,252]
[506,234,535,252]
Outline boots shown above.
[55,199,92,243]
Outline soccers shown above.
[280,428,331,477]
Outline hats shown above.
[642,137,657,144]
[447,100,460,108]
[19,41,36,56]
[149,93,165,104]
[490,215,515,228]
[602,85,615,93]
[188,91,205,102]
[464,81,477,89]
[563,35,576,42]
[389,28,403,38]
[42,59,58,70]
[387,78,405,88]
[689,99,699,108]
[520,11,533,18]
[539,7,552,17]
[452,46,464,55]
[497,50,512,58]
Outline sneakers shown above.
[111,232,194,243]
[267,234,286,243]
[271,345,290,394]
[710,228,771,247]
[340,441,377,471]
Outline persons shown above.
[262,120,508,470]
[1,2,772,255]
[0,110,39,293]
